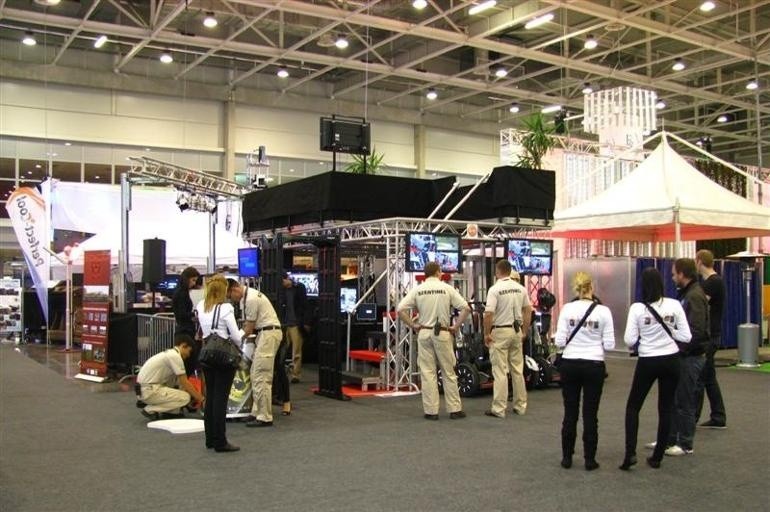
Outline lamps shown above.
[174,183,218,214]
[583,1,656,156]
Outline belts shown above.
[255,326,281,333]
[421,326,450,330]
[492,325,512,328]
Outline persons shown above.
[645,257,712,457]
[135,266,307,451]
[483,260,533,418]
[695,249,726,430]
[619,267,693,471]
[396,262,471,420]
[554,271,616,470]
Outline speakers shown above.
[143,239,166,284]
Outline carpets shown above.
[313,382,421,397]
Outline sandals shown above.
[282,401,291,416]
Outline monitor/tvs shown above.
[155,275,180,290]
[237,248,261,277]
[504,238,553,276]
[357,304,377,321]
[320,117,371,155]
[405,232,462,274]
[339,287,358,313]
[283,269,320,298]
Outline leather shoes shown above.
[214,442,240,452]
[561,459,599,470]
[142,409,182,421]
[485,409,497,417]
[424,411,466,419]
[240,415,273,427]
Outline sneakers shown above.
[292,378,299,383]
[701,419,728,429]
[620,441,693,470]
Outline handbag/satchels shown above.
[197,332,243,370]
